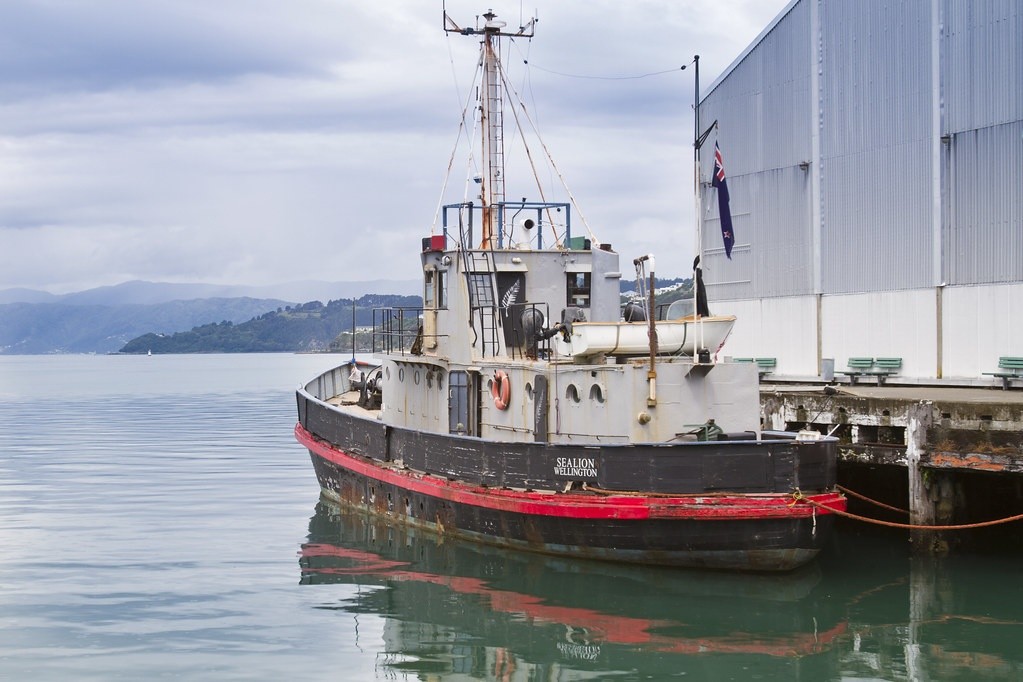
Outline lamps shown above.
[941,134,952,147]
[799,162,810,171]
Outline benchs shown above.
[844,357,902,387]
[734,358,777,383]
[981,357,1023,391]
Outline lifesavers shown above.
[492,371,510,411]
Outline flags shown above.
[712,140,735,261]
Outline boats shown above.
[294,7,852,573]
[553,315,737,357]
